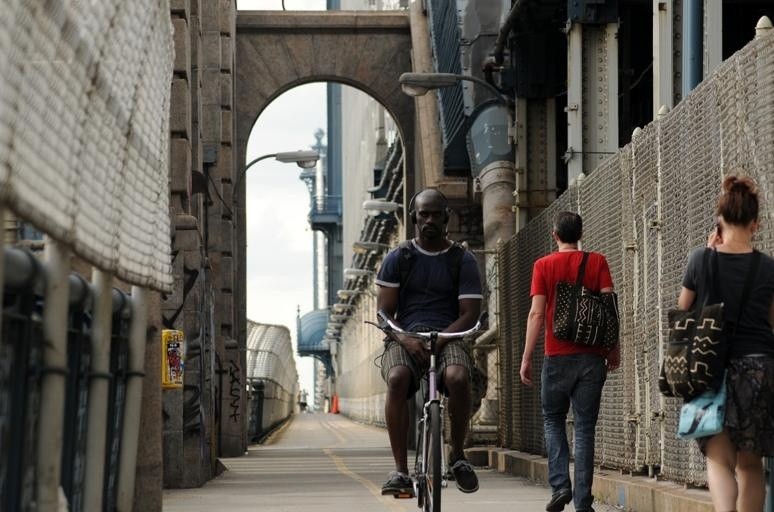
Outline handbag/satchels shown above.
[676,368,729,442]
[549,250,620,354]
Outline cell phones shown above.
[713,221,722,238]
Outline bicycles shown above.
[363,309,489,511]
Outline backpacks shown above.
[655,298,726,401]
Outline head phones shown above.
[408,187,450,225]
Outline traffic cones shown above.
[329,395,340,415]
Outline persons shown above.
[375,189,483,495]
[678,177,774,511]
[519,211,621,512]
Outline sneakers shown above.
[575,506,595,512]
[447,451,479,494]
[543,486,573,512]
[379,470,415,496]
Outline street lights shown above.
[398,72,511,110]
[239,150,323,191]
